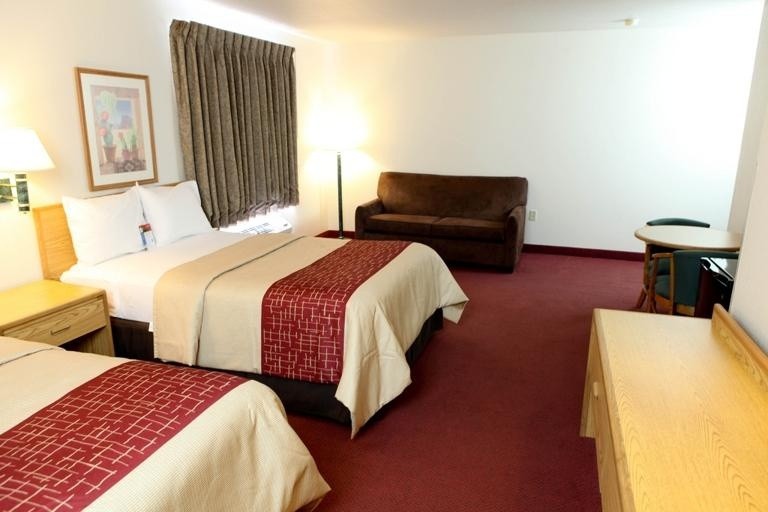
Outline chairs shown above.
[634,216,716,317]
[693,264,735,320]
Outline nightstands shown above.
[0,279,115,362]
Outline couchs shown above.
[355,170,529,274]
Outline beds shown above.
[0,335,332,512]
[31,180,470,439]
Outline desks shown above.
[635,224,743,316]
[579,303,768,512]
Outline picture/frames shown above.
[74,66,159,191]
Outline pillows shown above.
[137,180,212,249]
[60,183,146,269]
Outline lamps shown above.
[0,127,55,215]
[307,98,366,239]
